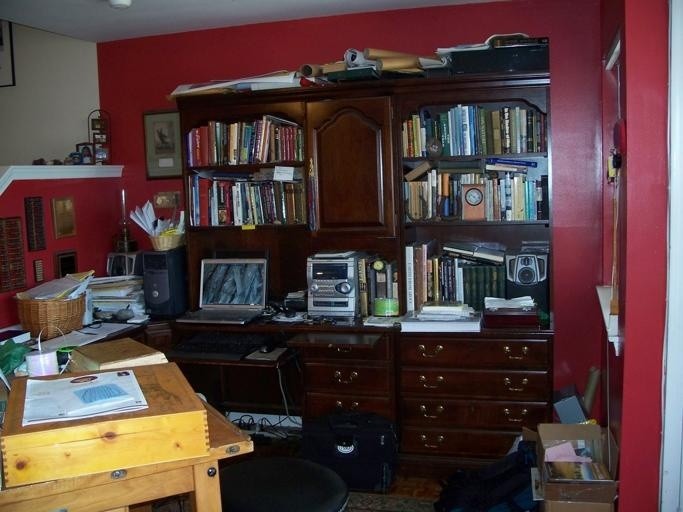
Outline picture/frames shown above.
[77,143,96,165]
[92,118,106,130]
[51,197,77,240]
[143,110,183,180]
[55,249,77,280]
[93,132,107,144]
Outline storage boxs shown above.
[536,423,619,512]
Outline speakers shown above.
[503,252,549,311]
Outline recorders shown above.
[307,252,367,319]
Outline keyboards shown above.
[168,330,267,363]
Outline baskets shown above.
[16,293,86,340]
[147,234,185,251]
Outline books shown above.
[401,103,548,158]
[403,160,549,224]
[401,234,507,314]
[184,114,305,162]
[186,159,314,230]
[358,256,401,319]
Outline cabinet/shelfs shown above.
[177,78,401,423]
[596,0,669,512]
[393,70,554,479]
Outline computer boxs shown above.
[142,249,189,321]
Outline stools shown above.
[220,457,350,512]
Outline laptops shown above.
[175,257,267,325]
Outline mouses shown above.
[258,338,277,354]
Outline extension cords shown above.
[233,423,289,439]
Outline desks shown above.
[0,376,255,512]
